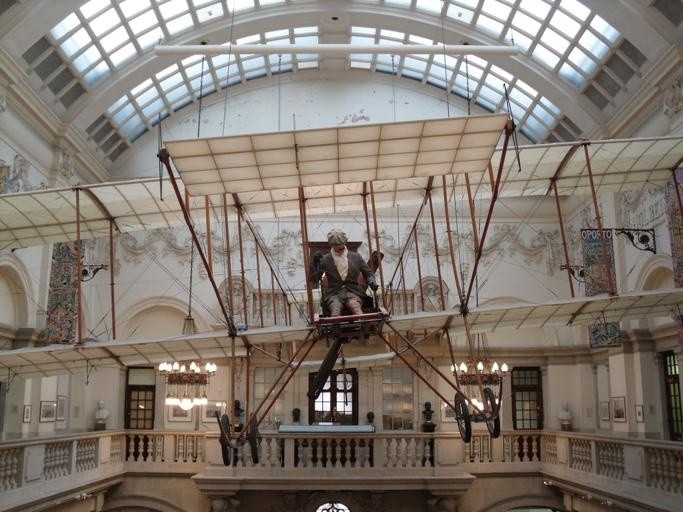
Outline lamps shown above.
[156,358,216,411]
[451,327,508,385]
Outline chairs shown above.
[320,271,374,316]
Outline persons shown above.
[422,401,434,419]
[229,399,246,415]
[307,227,378,332]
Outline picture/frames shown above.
[200,400,228,423]
[439,397,501,424]
[166,402,192,422]
[598,395,644,424]
[38,395,70,422]
[22,403,31,424]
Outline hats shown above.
[326,228,348,246]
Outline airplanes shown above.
[0,108,683,468]
